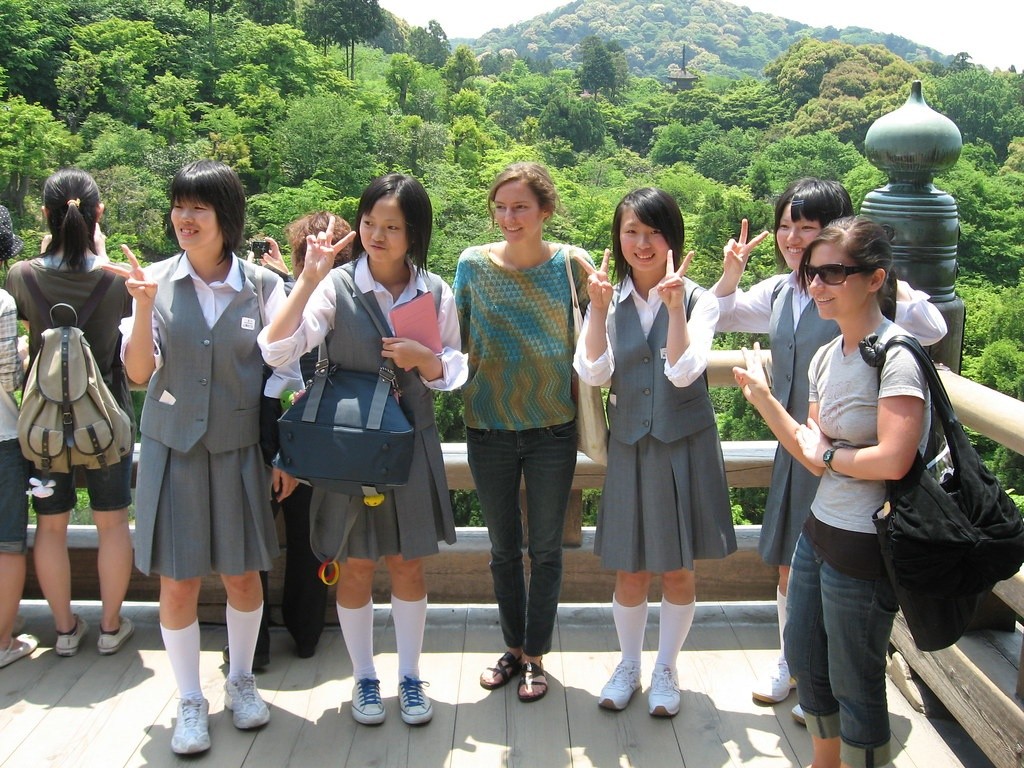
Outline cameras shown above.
[252,240,270,259]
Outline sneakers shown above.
[172,698,211,754]
[753,657,797,703]
[13,615,26,634]
[0,634,37,668]
[56,614,90,656]
[350,679,386,725]
[398,676,433,723]
[98,617,136,655]
[648,666,681,715]
[792,704,806,725]
[224,674,271,729]
[599,660,643,709]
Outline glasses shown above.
[801,264,866,285]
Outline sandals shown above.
[517,660,548,702]
[480,651,519,690]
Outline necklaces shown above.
[504,241,544,271]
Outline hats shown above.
[0,204,24,261]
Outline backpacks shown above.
[16,262,131,486]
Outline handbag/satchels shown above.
[858,333,1024,651]
[565,243,609,466]
[272,268,415,496]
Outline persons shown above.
[256,174,469,725]
[706,178,949,728]
[572,185,739,718]
[730,215,933,768]
[3,167,135,657]
[0,204,38,668]
[223,210,358,672]
[102,161,307,754]
[450,162,598,704]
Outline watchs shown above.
[823,447,842,473]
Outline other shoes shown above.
[223,646,270,672]
[281,634,314,658]
